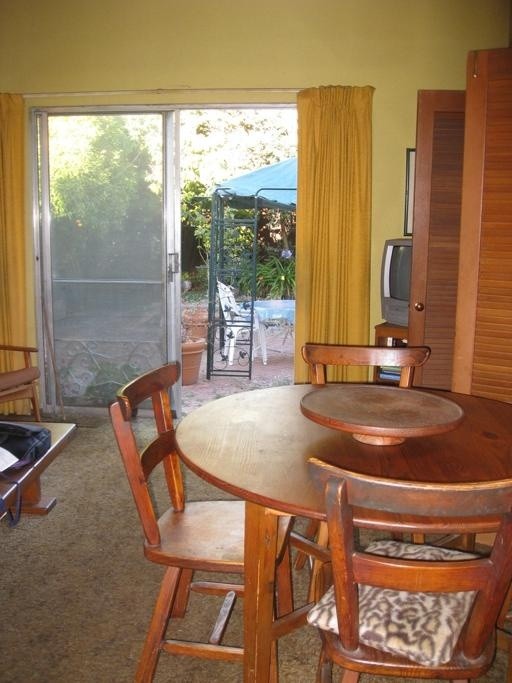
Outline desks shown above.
[241,299,295,345]
[1,420,79,515]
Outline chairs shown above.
[217,280,268,366]
[302,477,512,682]
[1,344,42,422]
[295,340,429,612]
[108,361,294,682]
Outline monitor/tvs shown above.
[380,238,412,328]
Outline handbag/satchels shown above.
[1,419,52,526]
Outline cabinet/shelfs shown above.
[372,322,408,383]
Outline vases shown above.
[181,308,221,385]
